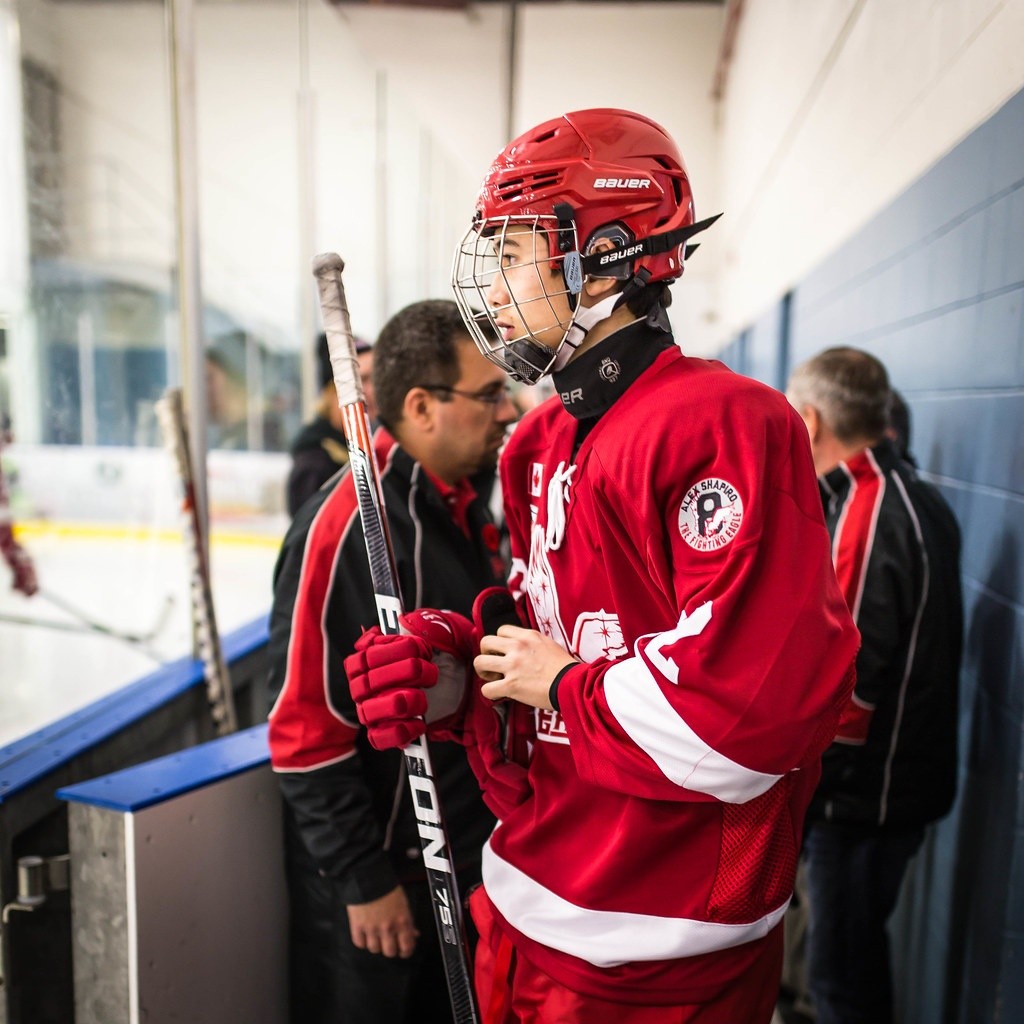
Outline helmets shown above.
[451,107,698,385]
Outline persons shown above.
[344,108,861,1023]
[264,298,524,1024]
[285,330,380,522]
[782,346,965,1024]
[0,463,39,597]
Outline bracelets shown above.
[549,662,581,712]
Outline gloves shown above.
[343,609,479,755]
[463,586,533,822]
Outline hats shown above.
[315,335,374,388]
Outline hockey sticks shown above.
[35,585,177,648]
[155,387,241,737]
[310,250,480,1024]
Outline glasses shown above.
[417,386,510,411]
